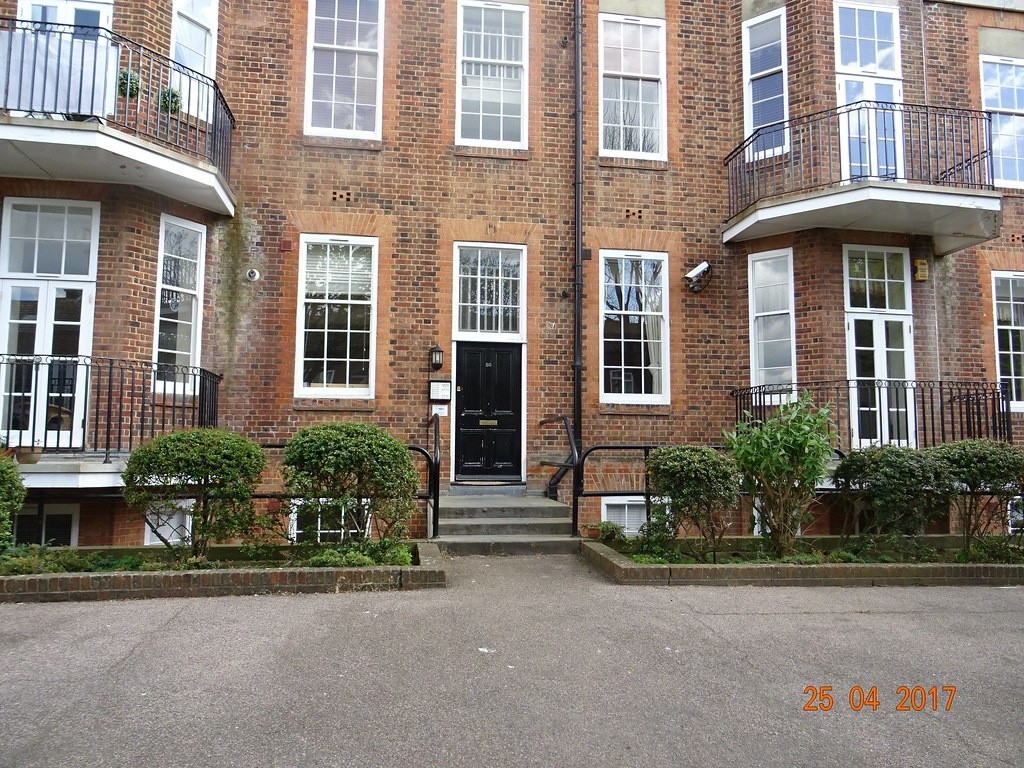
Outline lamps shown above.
[430,345,443,371]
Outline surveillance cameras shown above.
[684,261,708,284]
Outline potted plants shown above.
[16,439,42,464]
[0,434,15,461]
